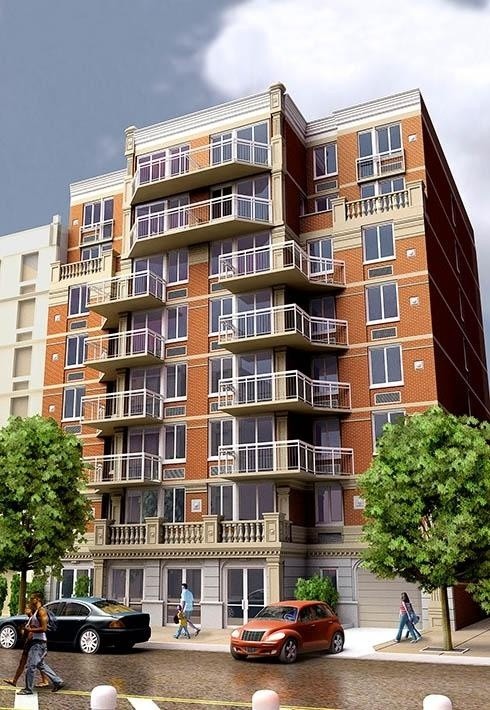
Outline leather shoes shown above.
[5,679,73,696]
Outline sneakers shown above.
[175,629,206,641]
